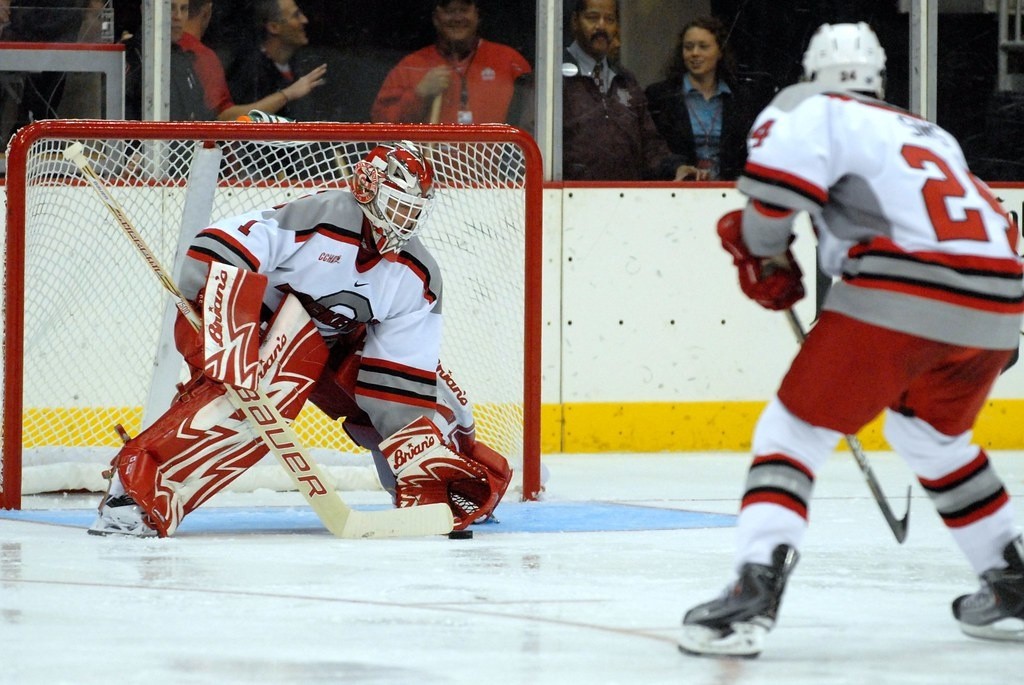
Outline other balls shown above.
[449,527,473,539]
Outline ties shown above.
[594,64,607,112]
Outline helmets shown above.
[349,140,434,256]
[803,22,885,99]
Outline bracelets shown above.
[277,88,289,103]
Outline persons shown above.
[367,0,533,179]
[500,0,711,182]
[89,139,516,534]
[226,0,346,180]
[643,20,753,181]
[608,22,642,93]
[172,0,327,149]
[673,19,1024,658]
[101,0,219,179]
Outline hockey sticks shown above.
[781,306,913,545]
[62,139,458,542]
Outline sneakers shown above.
[680,544,800,660]
[88,492,158,537]
[952,535,1024,643]
